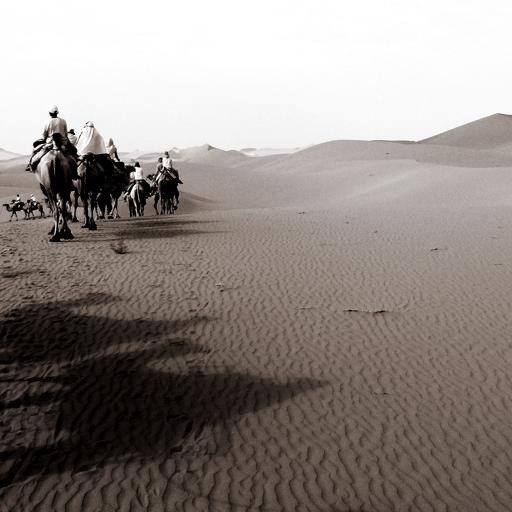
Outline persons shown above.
[124,162,151,202]
[105,137,124,175]
[74,121,106,171]
[25,105,81,171]
[152,157,167,182]
[27,194,37,213]
[9,194,21,211]
[67,129,78,145]
[162,151,179,180]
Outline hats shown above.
[68,130,76,134]
[49,105,58,113]
